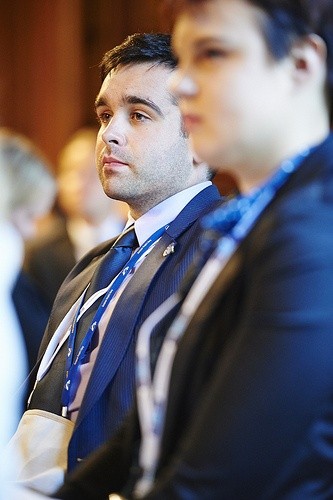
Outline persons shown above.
[10,31,235,500]
[22,0,333,500]
[1,117,142,500]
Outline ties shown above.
[28,223,140,421]
